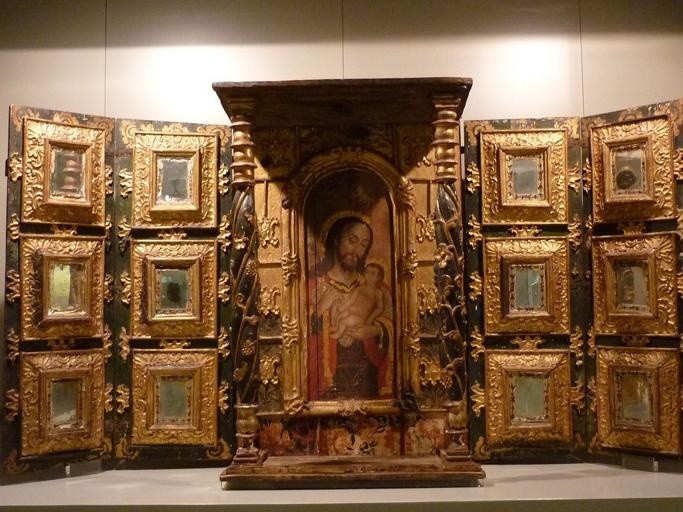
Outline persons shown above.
[327,262,385,341]
[303,214,394,401]
